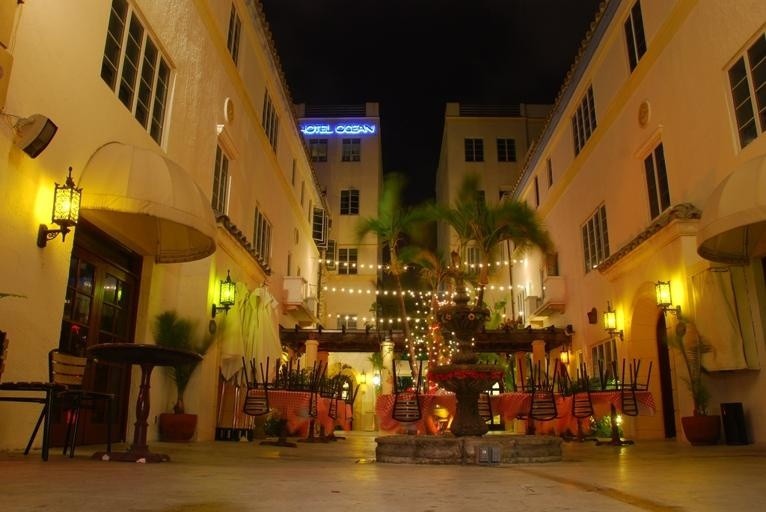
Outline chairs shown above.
[241,354,654,423]
[0,329,117,462]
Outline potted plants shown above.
[151,309,219,442]
[661,314,721,446]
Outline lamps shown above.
[654,279,682,321]
[37,166,83,248]
[211,269,236,319]
[601,300,624,341]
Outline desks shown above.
[87,342,204,464]
